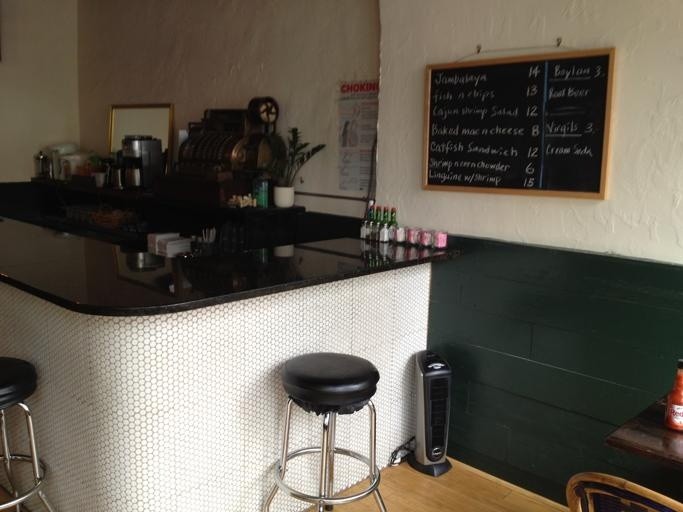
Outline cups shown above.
[96,173,105,188]
[396,226,448,249]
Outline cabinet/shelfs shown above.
[31,176,307,246]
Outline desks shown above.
[602,389,683,468]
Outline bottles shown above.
[360,239,396,268]
[252,172,270,208]
[358,200,397,243]
[396,246,446,262]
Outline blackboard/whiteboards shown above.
[422,48,615,200]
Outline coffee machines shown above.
[122,134,163,191]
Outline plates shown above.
[126,251,165,270]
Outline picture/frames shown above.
[107,102,175,162]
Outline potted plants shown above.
[273,127,327,209]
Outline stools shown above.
[262,351,389,512]
[0,356,56,510]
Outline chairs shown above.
[566,472,682,512]
